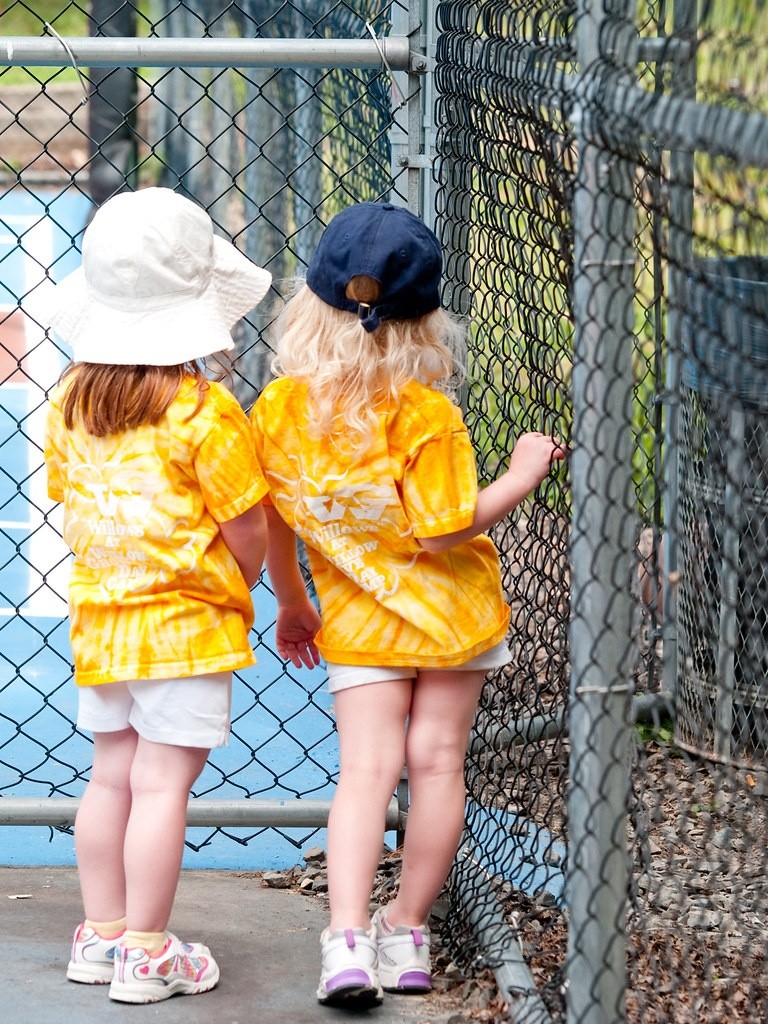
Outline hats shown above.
[306,202,443,329]
[41,188,271,366]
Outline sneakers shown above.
[371,901,432,992]
[65,923,125,983]
[317,926,384,1008]
[109,929,221,1005]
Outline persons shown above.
[252,200,567,1009]
[23,189,271,1005]
